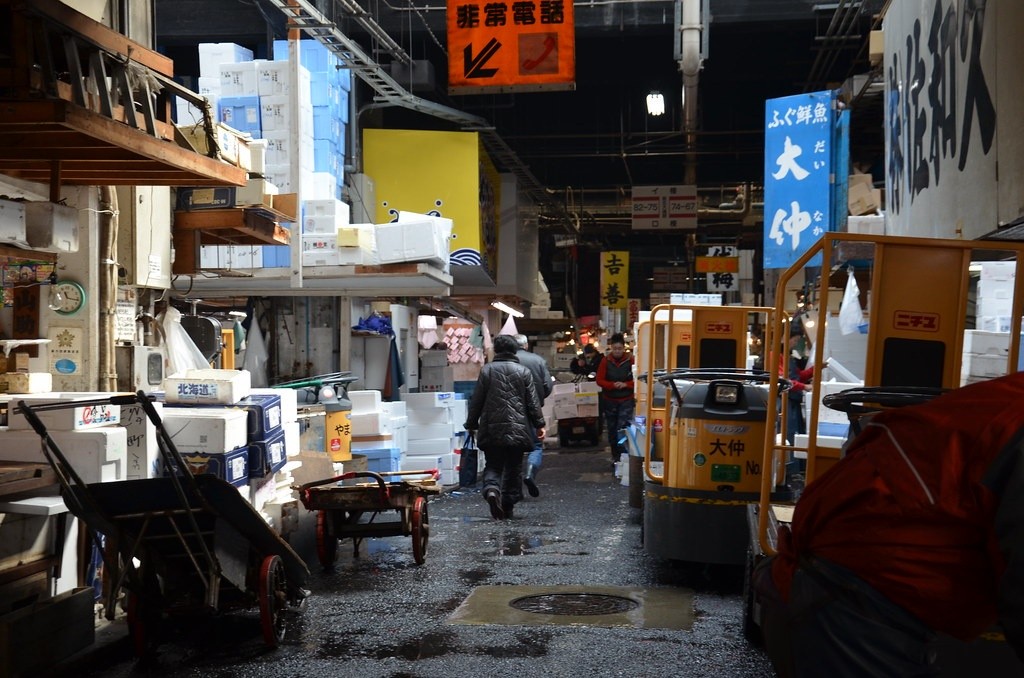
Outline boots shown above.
[524,464,541,497]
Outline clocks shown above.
[48,279,86,316]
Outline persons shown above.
[760,372,1024,678]
[749,323,829,482]
[463,334,547,520]
[570,343,605,442]
[514,335,552,502]
[596,333,634,466]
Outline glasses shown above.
[586,356,593,359]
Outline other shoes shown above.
[502,500,513,519]
[486,490,505,520]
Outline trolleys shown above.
[294,466,441,573]
[9,388,313,669]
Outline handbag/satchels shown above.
[459,435,478,488]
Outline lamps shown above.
[646,88,666,116]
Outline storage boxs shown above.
[650,266,687,311]
[826,28,884,113]
[0,367,485,534]
[961,329,1010,389]
[529,271,564,319]
[174,37,452,269]
[533,340,602,449]
[0,199,80,254]
[848,173,886,239]
[802,357,864,438]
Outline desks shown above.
[0,492,72,599]
